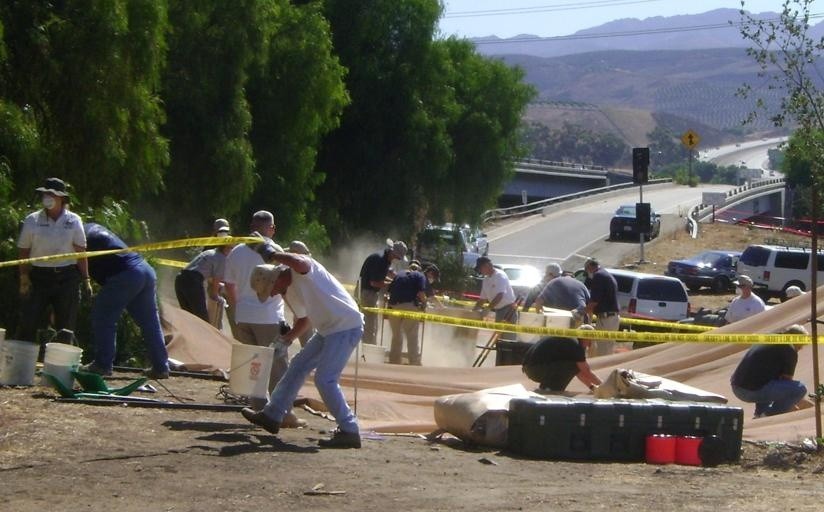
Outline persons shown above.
[584,259,620,357]
[177,210,307,428]
[17,177,93,341]
[240,233,365,448]
[535,270,591,324]
[730,325,807,418]
[522,325,602,398]
[471,256,518,325]
[354,238,441,366]
[78,222,171,380]
[723,274,766,325]
[521,261,563,312]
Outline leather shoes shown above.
[141,366,172,380]
[318,429,363,449]
[75,359,114,378]
[240,406,279,435]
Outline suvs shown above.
[733,242,824,304]
[417,218,492,268]
[565,265,692,324]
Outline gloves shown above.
[245,229,277,264]
[18,273,34,300]
[82,276,95,297]
[266,335,290,360]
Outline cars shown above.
[431,250,486,294]
[693,135,784,177]
[490,263,541,299]
[608,203,668,244]
[663,248,742,294]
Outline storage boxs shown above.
[491,334,538,367]
[503,388,745,469]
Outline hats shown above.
[731,273,756,288]
[34,177,75,203]
[249,261,285,306]
[385,236,410,264]
[473,255,492,271]
[214,218,230,233]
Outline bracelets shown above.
[590,385,593,390]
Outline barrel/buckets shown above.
[517,310,545,344]
[545,309,573,330]
[0,340,40,388]
[676,435,705,466]
[643,433,676,465]
[362,343,386,365]
[41,342,84,390]
[228,343,276,400]
